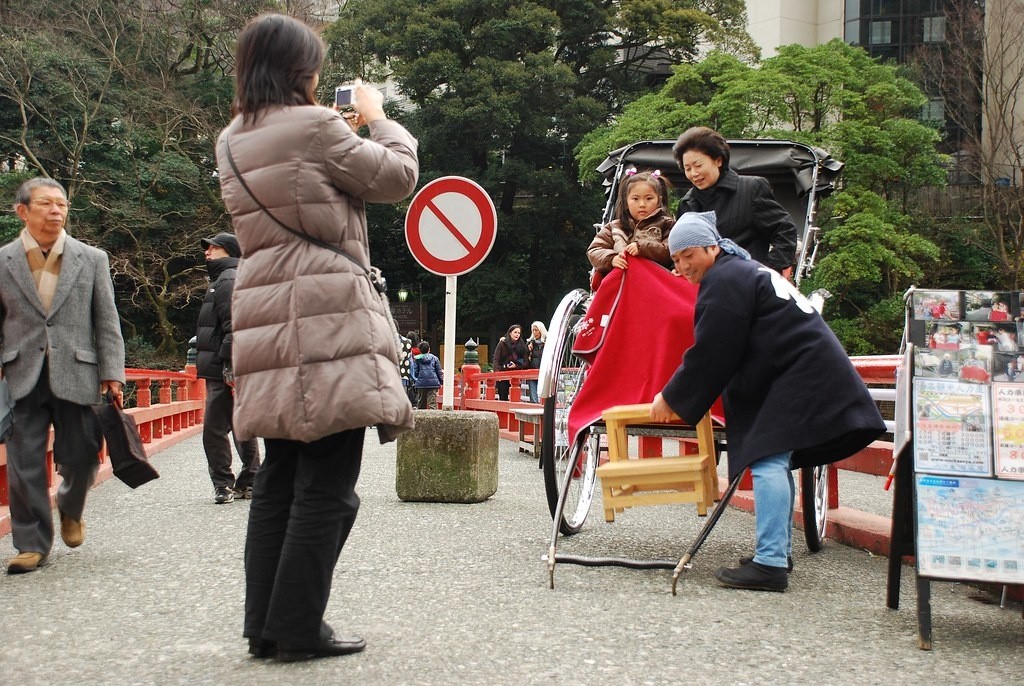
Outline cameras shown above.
[336,87,358,108]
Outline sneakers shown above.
[7,552,48,574]
[716,559,788,592]
[214,487,234,502]
[234,486,253,498]
[740,554,793,574]
[58,506,84,547]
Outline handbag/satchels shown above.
[97,388,159,489]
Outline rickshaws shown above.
[534,132,847,596]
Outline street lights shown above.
[396,281,424,341]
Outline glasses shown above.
[27,198,72,209]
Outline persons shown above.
[587,127,797,308]
[916,297,1024,383]
[650,212,870,591]
[494,321,549,403]
[0,177,126,575]
[214,12,419,663]
[195,233,261,504]
[393,319,443,410]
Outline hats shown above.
[201,231,242,258]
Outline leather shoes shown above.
[248,636,276,656]
[277,634,365,660]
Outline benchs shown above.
[510,408,569,458]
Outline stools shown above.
[596,396,720,522]
